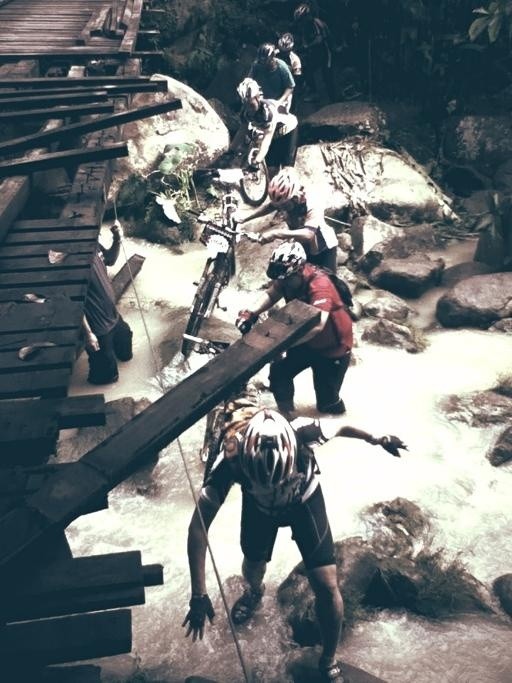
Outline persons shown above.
[236,239,356,422]
[215,74,301,179]
[178,403,412,683]
[294,1,339,106]
[246,42,297,113]
[234,176,342,277]
[271,32,302,82]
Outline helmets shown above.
[241,409,299,512]
[278,32,294,52]
[268,166,302,209]
[293,4,310,20]
[236,78,263,103]
[265,241,306,279]
[256,41,277,64]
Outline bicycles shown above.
[179,207,263,361]
[222,124,271,206]
[182,311,263,487]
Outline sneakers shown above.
[322,662,348,683]
[231,585,266,624]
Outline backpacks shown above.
[306,265,354,312]
